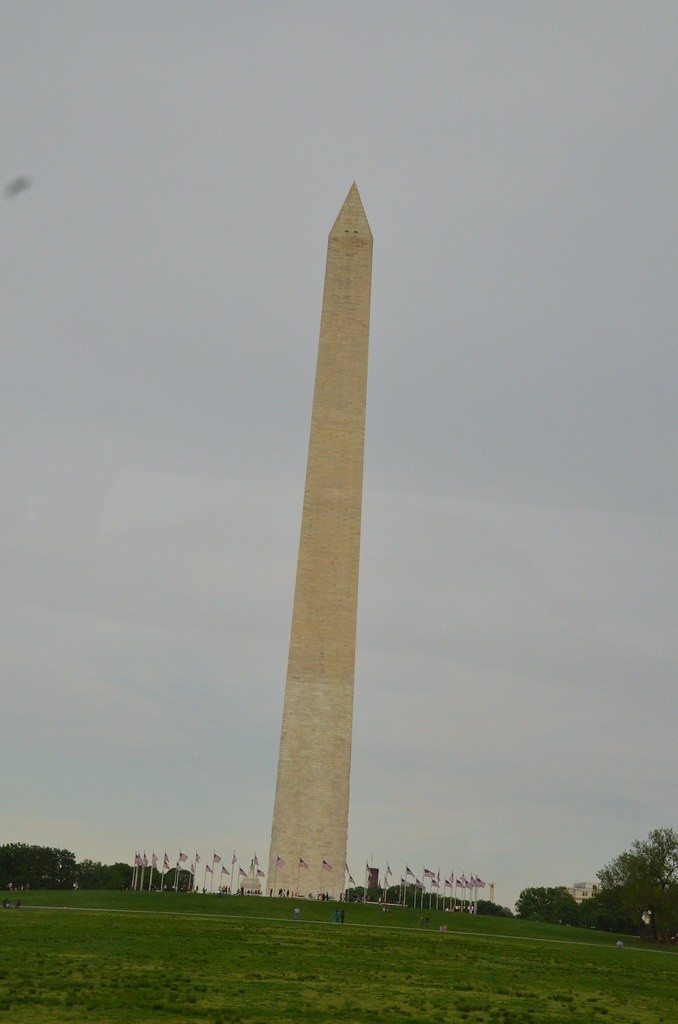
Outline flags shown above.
[135,853,265,878]
[345,864,486,889]
[322,861,332,872]
[277,858,285,867]
[300,859,308,869]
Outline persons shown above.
[380,907,391,913]
[217,885,231,896]
[150,884,207,894]
[333,910,345,925]
[4,898,20,908]
[420,915,429,927]
[445,904,474,914]
[339,893,370,903]
[617,939,624,951]
[439,924,447,931]
[294,906,299,920]
[236,887,260,896]
[270,888,333,901]
[72,881,79,890]
[379,898,404,906]
